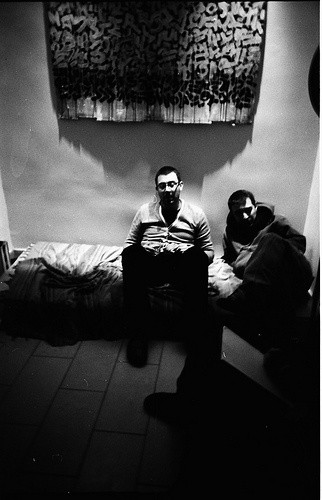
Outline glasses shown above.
[157,182,180,189]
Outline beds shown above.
[0,241,242,309]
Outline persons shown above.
[219,191,314,354]
[119,165,215,362]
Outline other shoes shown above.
[217,286,254,313]
[126,340,149,366]
